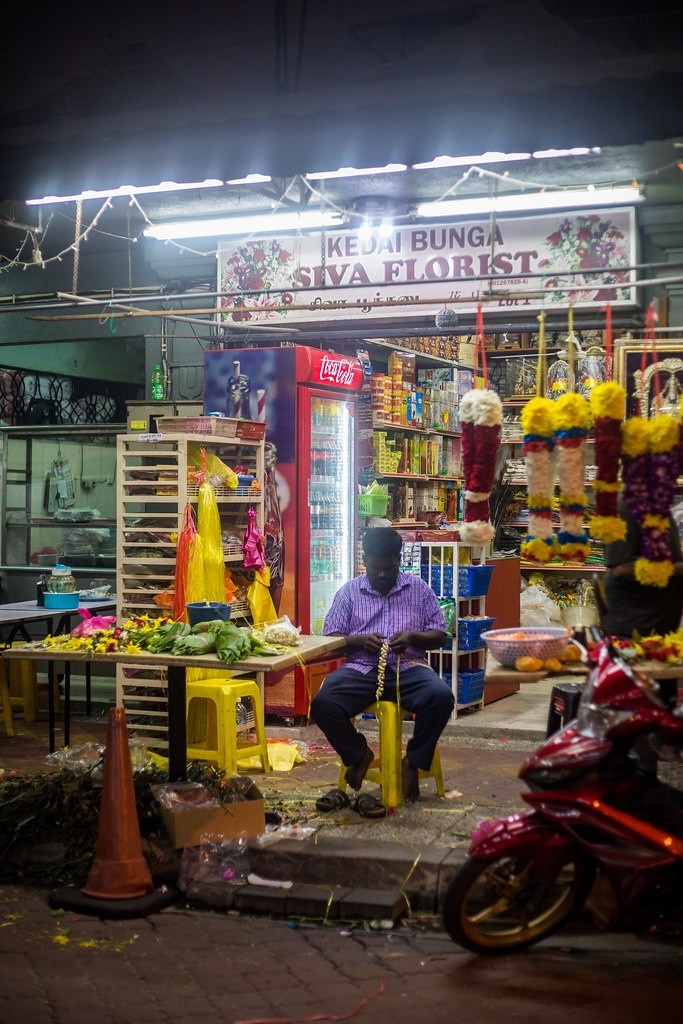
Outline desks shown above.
[1,634,347,782]
[0,591,118,754]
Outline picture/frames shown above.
[613,338,683,489]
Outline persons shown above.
[311,528,455,799]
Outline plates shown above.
[79,598,109,602]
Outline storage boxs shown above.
[419,565,495,706]
[373,351,473,515]
[158,776,265,850]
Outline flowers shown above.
[608,621,683,667]
[25,611,176,655]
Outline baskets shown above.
[437,667,486,705]
[236,420,267,440]
[153,417,237,438]
[479,627,575,668]
[442,617,496,651]
[360,493,390,516]
[420,564,496,596]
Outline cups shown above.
[90,578,108,589]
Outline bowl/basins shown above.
[35,554,57,566]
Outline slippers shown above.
[350,794,386,818]
[316,789,350,812]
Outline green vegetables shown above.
[129,619,284,665]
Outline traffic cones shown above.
[48,707,168,918]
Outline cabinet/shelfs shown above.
[116,434,265,749]
[472,555,521,706]
[500,401,606,573]
[359,338,483,528]
[417,542,486,722]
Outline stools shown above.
[185,678,270,780]
[337,699,445,810]
[547,682,585,740]
[0,641,62,722]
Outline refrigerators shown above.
[204,346,364,726]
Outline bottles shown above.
[151,365,164,401]
[264,442,285,617]
[313,600,326,636]
[311,398,343,583]
[36,574,47,606]
[225,374,251,421]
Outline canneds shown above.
[372,361,403,425]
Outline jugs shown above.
[46,564,77,594]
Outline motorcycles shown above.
[442,573,683,957]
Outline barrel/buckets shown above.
[186,602,232,628]
